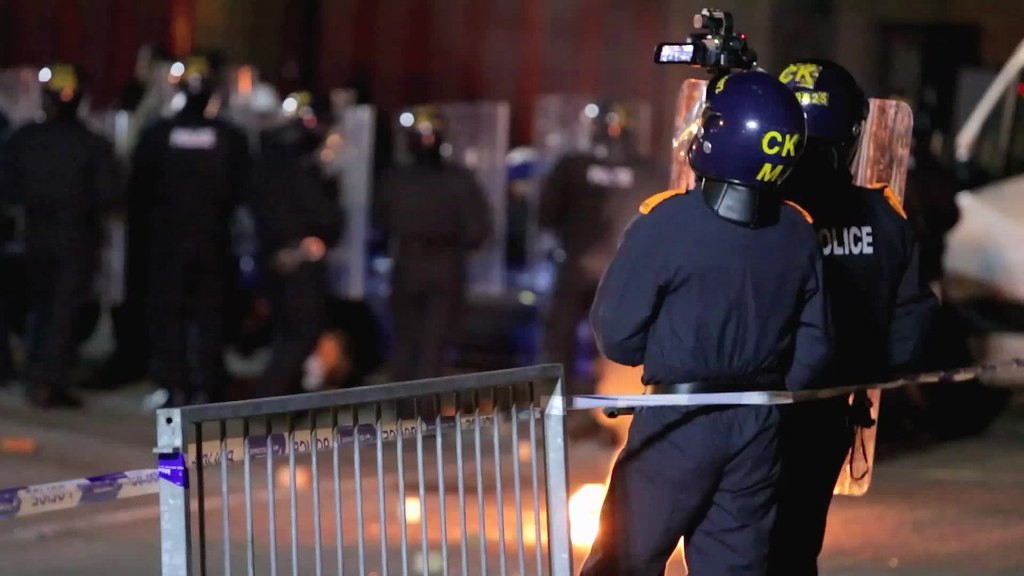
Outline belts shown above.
[646,380,775,396]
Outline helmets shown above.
[778,61,870,174]
[583,101,643,152]
[403,105,445,150]
[169,55,213,117]
[40,65,77,114]
[688,71,807,225]
[262,92,330,155]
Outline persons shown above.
[251,84,385,414]
[1,65,125,408]
[538,97,668,404]
[376,106,520,419]
[578,72,832,576]
[120,44,265,408]
[769,56,938,576]
